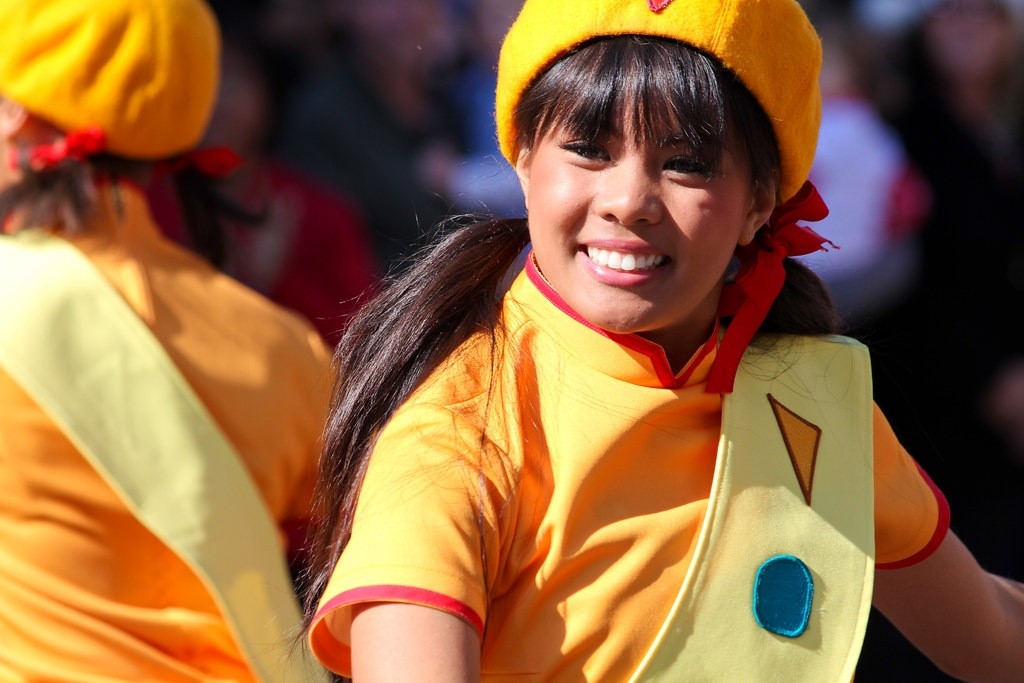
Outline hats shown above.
[0,0,221,157]
[494,0,823,204]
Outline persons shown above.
[308,0,1024,683]
[201,0,1024,683]
[0,0,339,683]
